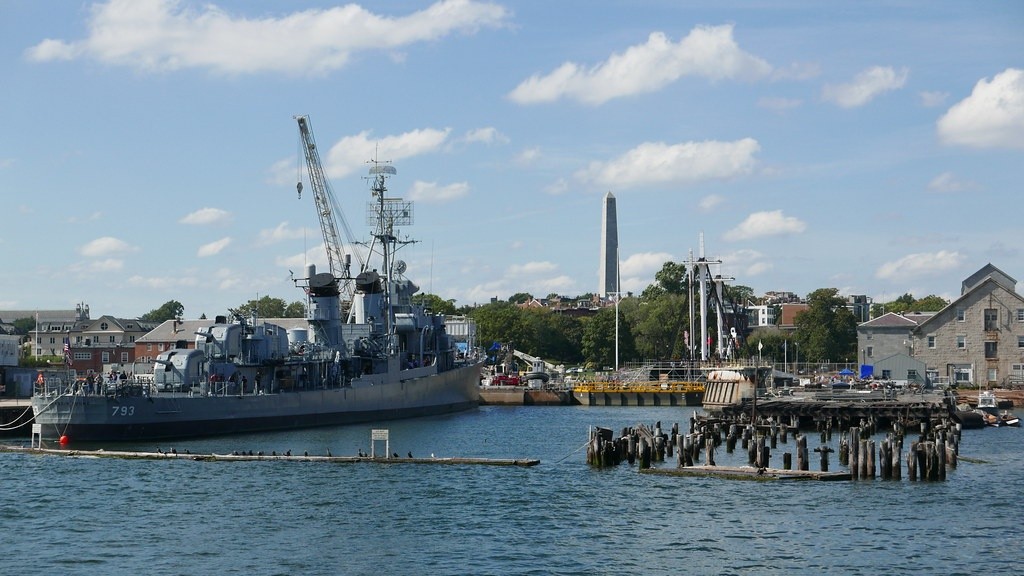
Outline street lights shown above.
[687,248,698,361]
[34,306,40,364]
[862,348,867,365]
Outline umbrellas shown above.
[838,369,855,375]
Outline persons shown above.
[83,370,104,395]
[241,376,248,393]
[149,366,154,372]
[254,372,261,394]
[107,370,127,385]
[209,371,219,396]
[36,371,45,395]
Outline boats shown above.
[982,411,1001,427]
[30,113,488,445]
[999,412,1019,425]
[973,372,999,415]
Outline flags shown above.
[64,337,70,352]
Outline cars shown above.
[814,366,829,373]
[565,366,585,373]
[799,367,812,375]
[602,365,614,371]
